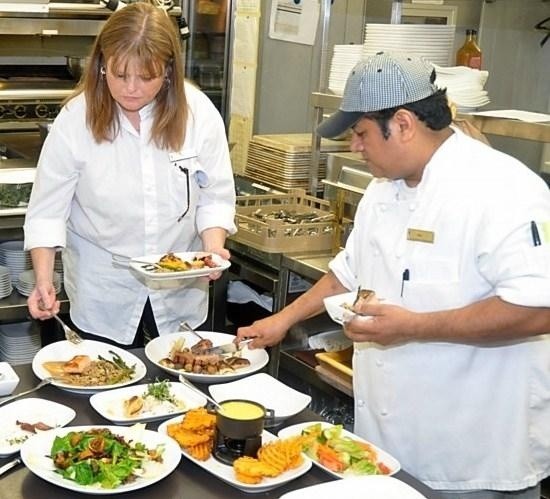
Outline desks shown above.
[0,344,443,499]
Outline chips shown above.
[232,439,303,484]
[167,407,216,461]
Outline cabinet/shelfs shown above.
[209,225,281,373]
[310,0,550,211]
[1,1,229,333]
[272,254,355,433]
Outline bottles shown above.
[457,29,482,69]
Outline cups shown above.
[213,400,275,467]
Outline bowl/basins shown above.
[322,292,399,327]
[0,359,19,397]
[66,53,90,82]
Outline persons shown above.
[233,50,550,499]
[23,3,237,348]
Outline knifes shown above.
[196,337,255,356]
[0,456,22,476]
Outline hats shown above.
[315,52,440,139]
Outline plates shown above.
[1,396,77,458]
[145,330,270,384]
[0,241,64,299]
[32,338,147,395]
[129,251,232,280]
[207,372,312,428]
[327,22,456,107]
[20,426,182,495]
[280,474,425,498]
[427,62,490,112]
[88,382,208,425]
[279,419,401,479]
[159,409,314,494]
[0,321,42,366]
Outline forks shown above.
[39,305,84,345]
[1,376,72,406]
